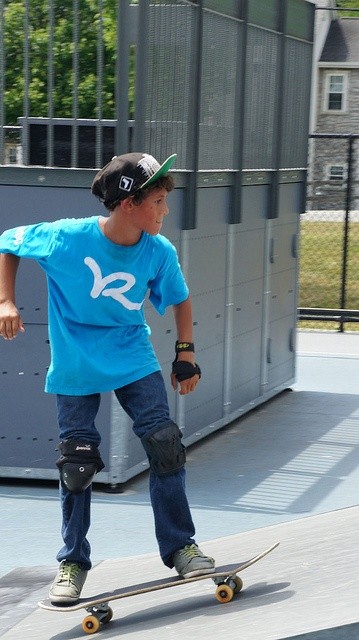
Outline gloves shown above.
[173,340,201,382]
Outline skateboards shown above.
[38,542,280,633]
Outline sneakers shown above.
[172,543,216,578]
[48,561,87,605]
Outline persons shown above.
[0,150,217,604]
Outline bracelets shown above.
[178,342,194,352]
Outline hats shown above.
[91,152,178,207]
